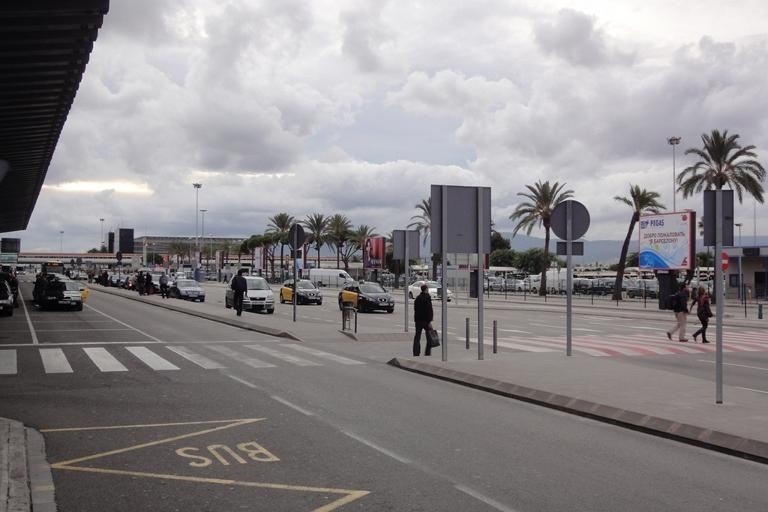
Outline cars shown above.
[484,267,715,299]
[226,274,394,313]
[408,281,452,302]
[0,261,88,316]
[93,264,205,302]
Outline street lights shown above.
[193,184,202,246]
[100,219,104,240]
[735,223,744,298]
[200,210,207,236]
[667,137,681,213]
[153,242,156,264]
[60,231,64,249]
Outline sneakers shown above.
[703,340,710,343]
[667,332,672,340]
[692,335,696,341]
[679,339,688,342]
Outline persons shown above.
[690,287,697,300]
[412,285,434,357]
[146,271,152,295]
[230,269,249,316]
[159,271,169,299]
[364,239,372,265]
[690,287,713,344]
[665,282,689,342]
[136,271,145,295]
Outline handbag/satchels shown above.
[667,295,681,310]
[428,329,440,346]
[700,295,710,317]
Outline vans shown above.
[302,269,353,288]
